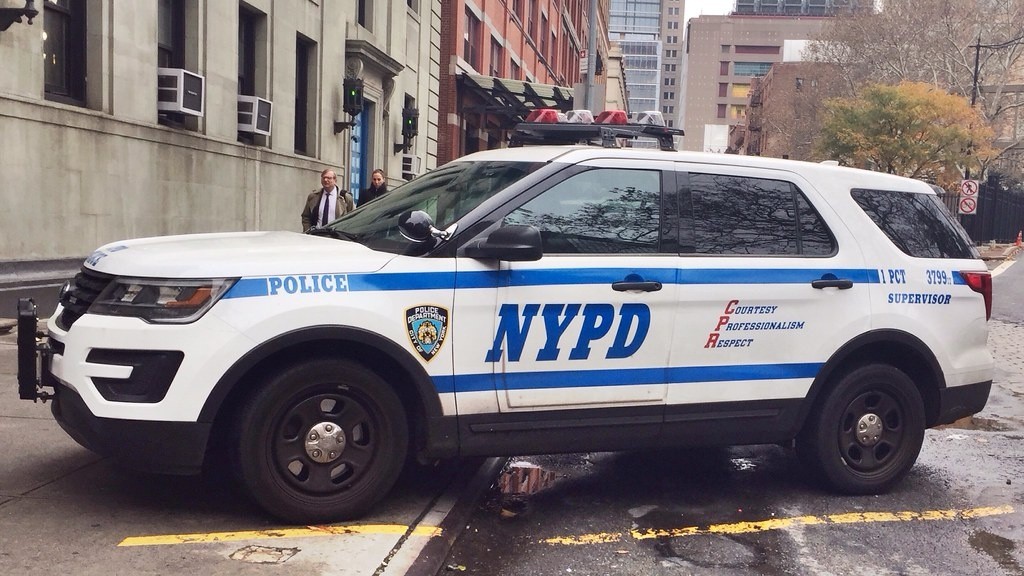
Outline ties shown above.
[322,193,330,227]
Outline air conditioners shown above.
[238,95,271,139]
[157,67,205,118]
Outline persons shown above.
[302,169,353,232]
[356,169,389,208]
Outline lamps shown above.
[334,77,364,136]
[394,108,419,156]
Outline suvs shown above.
[12,107,997,524]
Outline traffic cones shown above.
[1013,230,1023,246]
